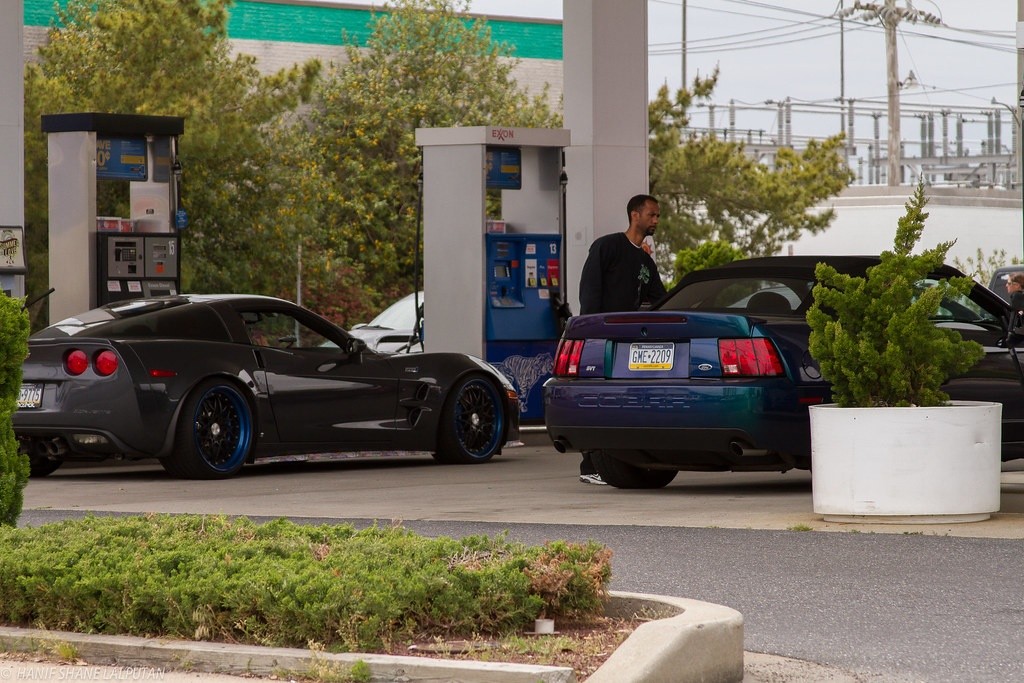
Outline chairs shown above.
[747,290,791,313]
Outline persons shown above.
[1005,273,1024,311]
[578,193,670,486]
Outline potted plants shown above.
[806,171,1002,523]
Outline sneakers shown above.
[579,473,607,485]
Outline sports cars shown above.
[10,292,522,480]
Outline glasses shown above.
[1006,280,1017,286]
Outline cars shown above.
[542,253,1024,489]
[317,290,425,352]
